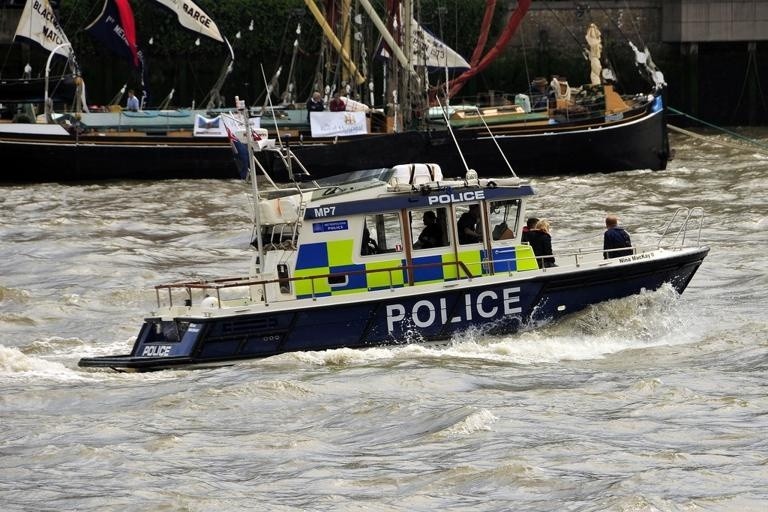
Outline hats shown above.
[526,217,539,228]
[605,216,618,226]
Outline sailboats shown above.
[0,0,675,185]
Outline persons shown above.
[306,91,345,121]
[602,214,633,260]
[361,230,379,255]
[126,90,139,113]
[415,205,556,269]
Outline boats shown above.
[77,185,710,372]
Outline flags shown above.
[224,121,249,182]
